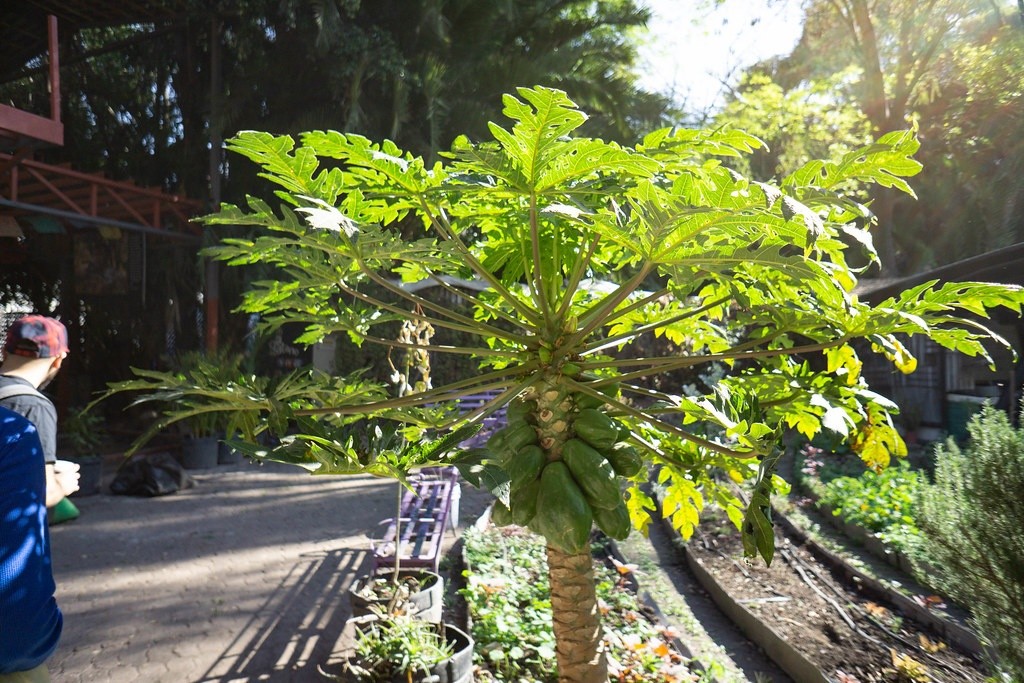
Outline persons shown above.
[0,314,80,683]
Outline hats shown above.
[5,315,70,358]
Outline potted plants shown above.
[58,407,111,498]
[341,303,475,683]
[160,341,267,468]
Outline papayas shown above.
[485,376,642,555]
